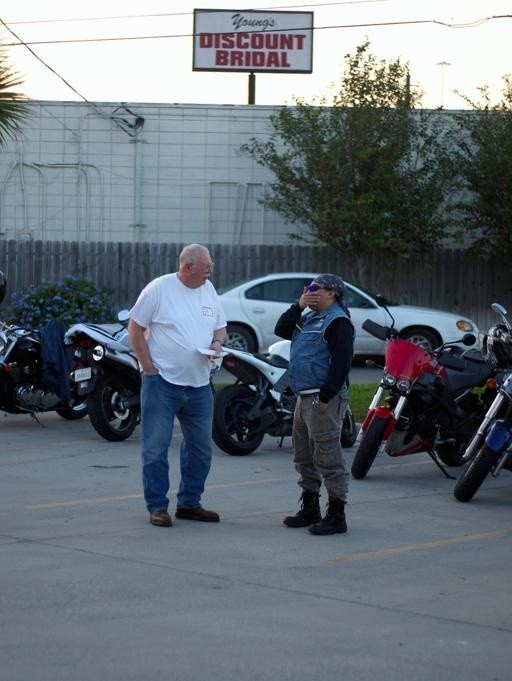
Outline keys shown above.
[311,392,320,409]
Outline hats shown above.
[316,273,351,317]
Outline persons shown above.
[275,274,356,537]
[126,244,227,527]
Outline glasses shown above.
[193,262,215,271]
[306,285,324,293]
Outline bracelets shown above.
[211,339,222,344]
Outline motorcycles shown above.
[64,309,154,442]
[450,303,511,503]
[209,331,362,457]
[349,293,479,476]
[0,280,93,419]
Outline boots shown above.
[284,492,322,526]
[309,499,347,535]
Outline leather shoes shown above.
[150,512,172,527]
[175,508,220,523]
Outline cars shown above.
[214,270,479,360]
